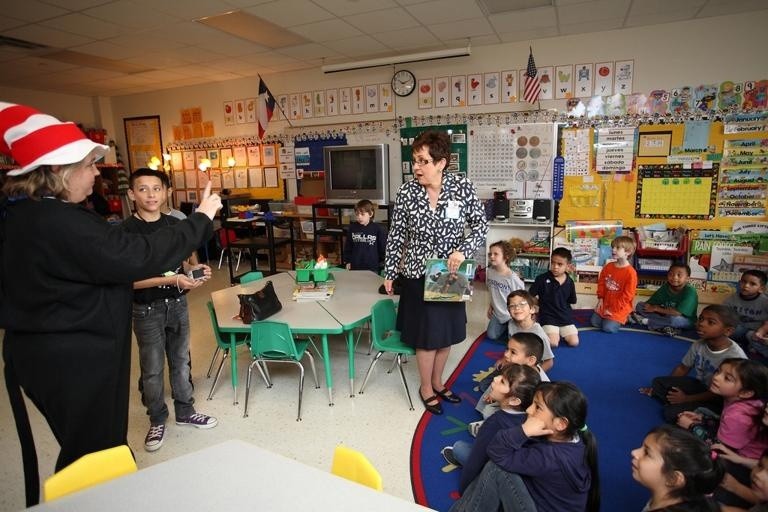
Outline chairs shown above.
[330,443,382,493]
[41,443,138,505]
[203,266,416,421]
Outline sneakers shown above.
[664,326,681,337]
[144,424,165,452]
[442,446,461,467]
[469,422,481,438]
[176,413,218,430]
[631,312,645,326]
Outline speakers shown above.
[493,190,509,223]
[533,198,552,224]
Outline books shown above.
[634,223,688,251]
[687,230,768,295]
[292,285,335,303]
[424,258,475,303]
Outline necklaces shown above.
[136,212,148,224]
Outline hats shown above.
[0,101,110,178]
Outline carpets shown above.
[409,307,705,510]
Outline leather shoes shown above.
[419,387,443,415]
[433,387,461,403]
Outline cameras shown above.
[187,267,207,280]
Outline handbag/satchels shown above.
[237,281,283,324]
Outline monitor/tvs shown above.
[323,144,390,206]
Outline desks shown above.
[17,437,436,511]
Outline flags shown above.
[522,50,542,104]
[256,78,277,141]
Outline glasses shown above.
[508,301,528,310]
[411,160,433,166]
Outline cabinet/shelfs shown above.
[485,220,553,283]
[311,202,394,275]
[222,215,341,287]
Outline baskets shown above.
[300,220,327,234]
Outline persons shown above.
[630,269,766,511]
[449,380,600,511]
[632,262,698,337]
[136,172,204,417]
[448,234,578,499]
[342,198,387,276]
[1,150,226,475]
[590,235,638,333]
[115,167,220,454]
[136,169,200,267]
[381,129,491,416]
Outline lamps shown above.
[320,40,471,73]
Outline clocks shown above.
[390,69,416,97]
